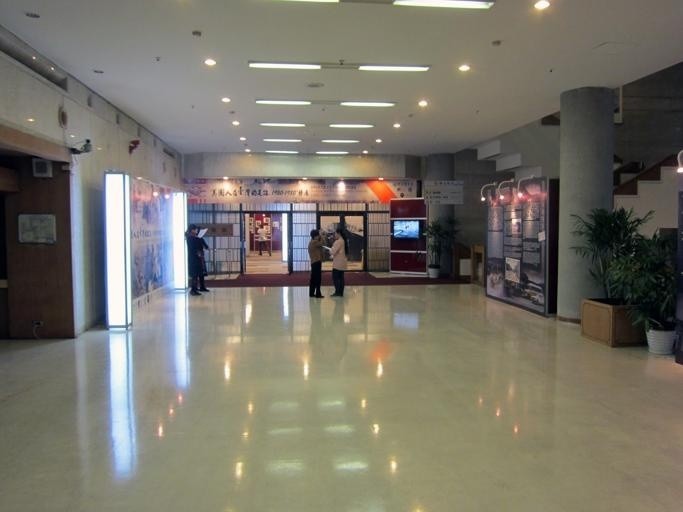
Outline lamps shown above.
[480,175,536,202]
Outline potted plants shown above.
[565,202,682,355]
[414,215,464,279]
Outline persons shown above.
[257,225,271,256]
[195,225,210,292]
[186,223,202,295]
[308,229,327,298]
[326,228,348,296]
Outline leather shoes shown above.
[310,295,324,298]
[331,292,343,296]
[190,288,209,296]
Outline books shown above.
[199,228,208,239]
[322,245,330,250]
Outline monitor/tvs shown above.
[391,219,423,241]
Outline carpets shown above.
[188,271,471,288]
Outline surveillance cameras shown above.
[83,139,92,152]
[129,139,140,146]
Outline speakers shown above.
[31,158,53,178]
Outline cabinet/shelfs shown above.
[484,176,560,318]
